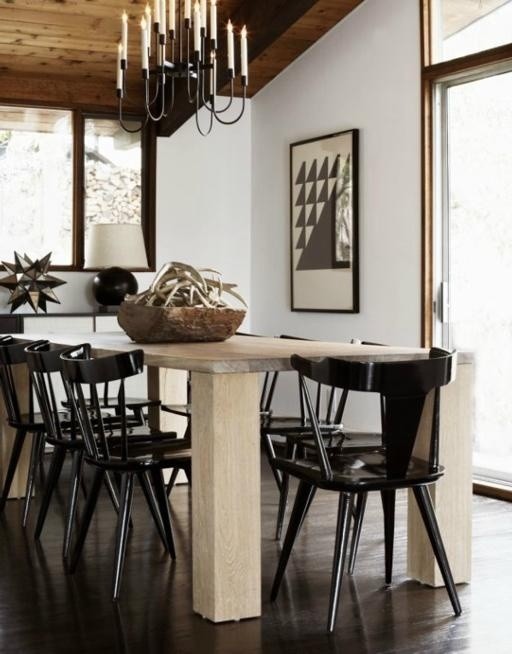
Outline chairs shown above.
[258,333,333,440]
[0,334,110,514]
[22,339,94,543]
[62,343,182,602]
[269,348,463,633]
[165,331,261,499]
[259,340,389,540]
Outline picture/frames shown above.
[289,129,359,314]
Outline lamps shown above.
[81,222,151,313]
[116,0,249,138]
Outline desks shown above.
[8,330,376,624]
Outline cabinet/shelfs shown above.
[0,311,190,486]
